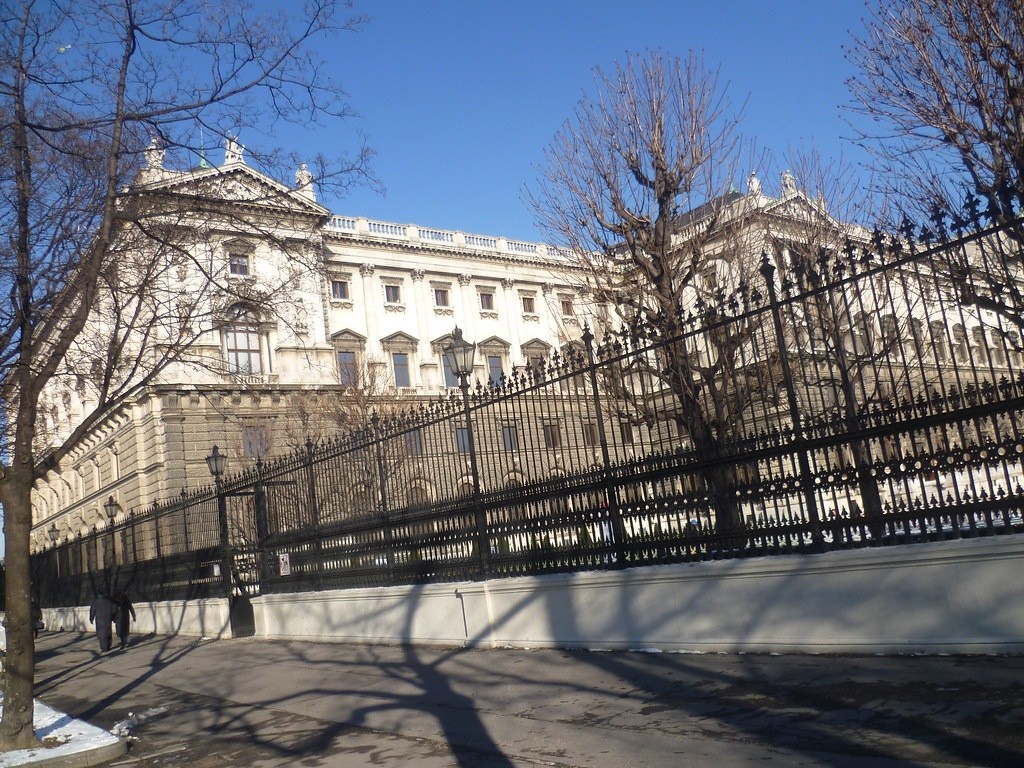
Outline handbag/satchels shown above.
[36,620,44,629]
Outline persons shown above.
[113,586,136,645]
[32,602,42,638]
[89,590,115,653]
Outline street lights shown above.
[46,521,63,607]
[102,495,121,601]
[442,324,497,583]
[201,443,237,599]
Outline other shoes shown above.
[102,647,110,652]
